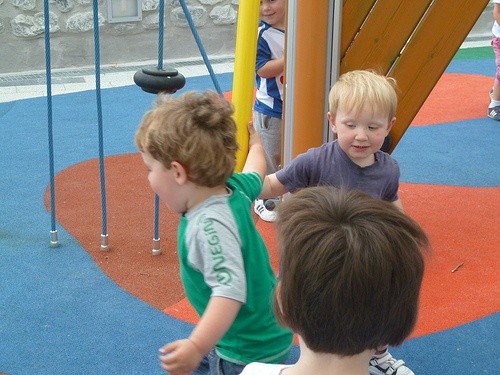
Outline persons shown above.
[255,70,415,375]
[239,186,433,375]
[135,90,294,375]
[253,0,285,221]
[487,0,500,121]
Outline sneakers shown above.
[488,106,500,121]
[489,90,494,100]
[369,348,415,375]
[254,198,278,222]
